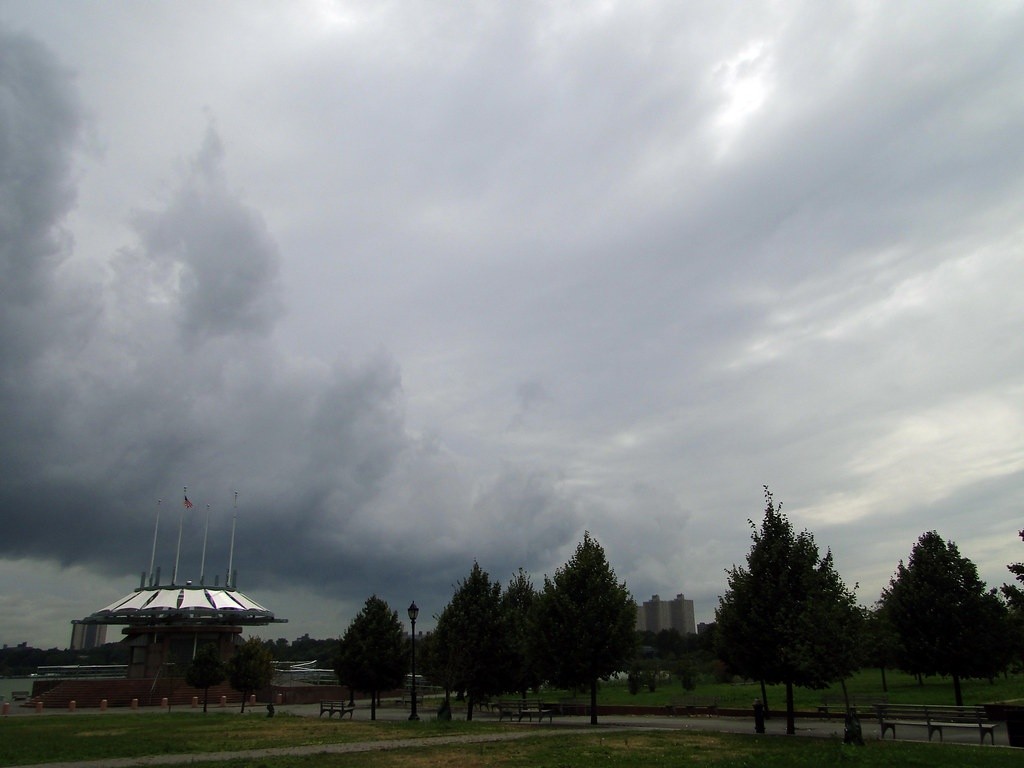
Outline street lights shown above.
[407,600,421,722]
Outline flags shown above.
[185,496,193,509]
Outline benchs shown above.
[667,695,721,718]
[319,699,353,720]
[872,701,998,746]
[495,699,553,725]
